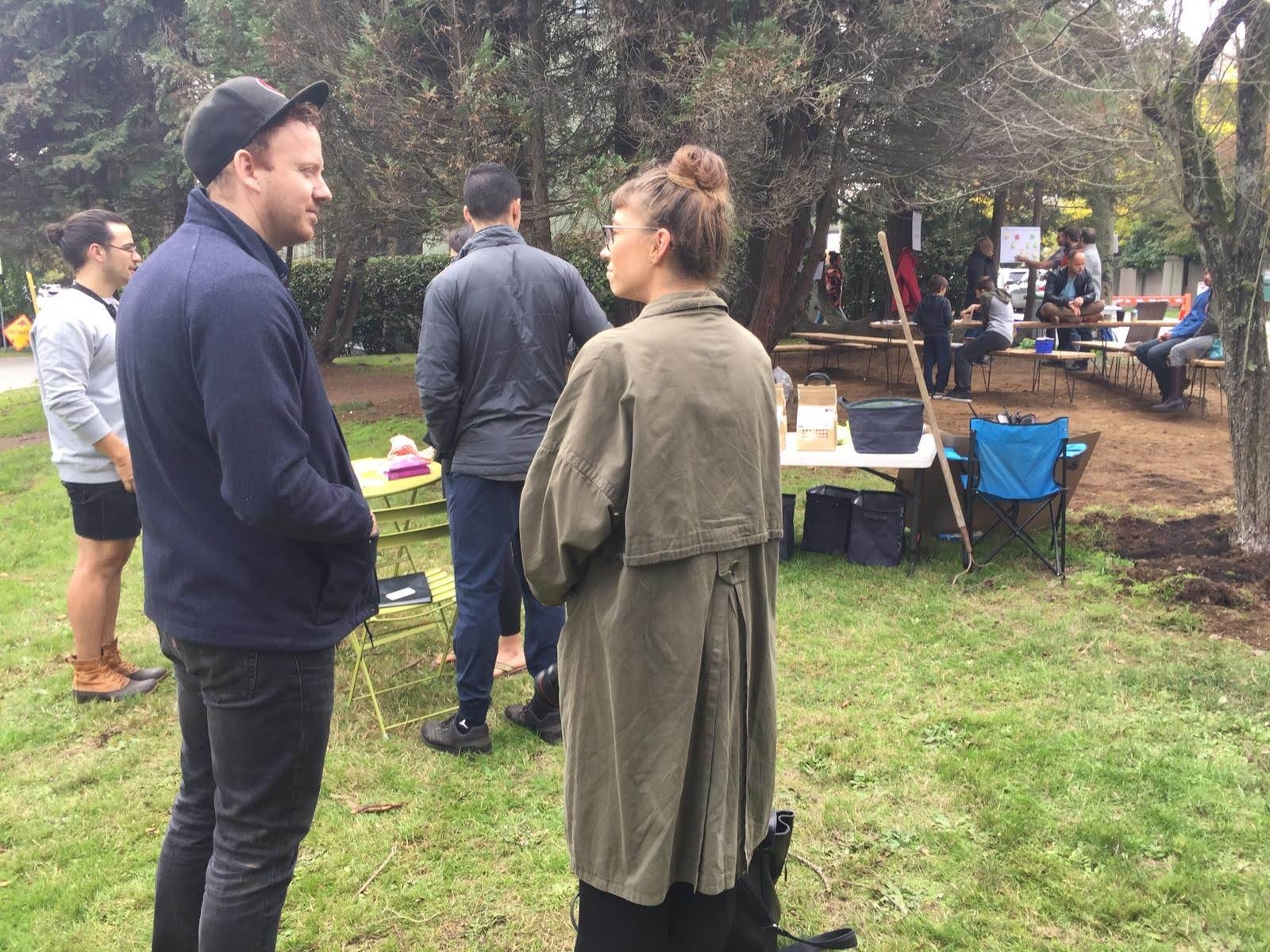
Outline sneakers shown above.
[419,711,494,757]
[506,701,564,746]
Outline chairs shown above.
[347,499,459,741]
[1105,301,1168,358]
[942,416,1089,581]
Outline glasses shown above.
[600,224,677,253]
[94,240,138,254]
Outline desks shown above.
[778,425,936,579]
[348,454,449,635]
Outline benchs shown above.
[772,300,1225,407]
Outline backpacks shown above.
[1098,326,1118,342]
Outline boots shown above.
[1151,363,1187,412]
[66,636,169,705]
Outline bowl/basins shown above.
[963,336,974,343]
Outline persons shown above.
[522,143,789,951]
[109,71,382,952]
[946,279,1015,403]
[26,211,171,703]
[814,251,847,326]
[1012,223,1103,371]
[964,236,998,364]
[410,157,628,751]
[1136,261,1240,411]
[1037,250,1096,371]
[912,275,957,402]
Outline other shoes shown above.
[929,389,949,399]
[1048,360,1064,367]
[1158,376,1190,404]
[942,387,972,402]
[1065,362,1087,371]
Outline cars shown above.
[997,268,1049,313]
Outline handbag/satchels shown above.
[780,492,797,561]
[1208,337,1223,361]
[848,490,906,566]
[774,378,788,450]
[728,809,858,952]
[799,484,860,556]
[839,396,925,455]
[796,371,838,452]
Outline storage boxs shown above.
[797,384,836,452]
[776,385,788,449]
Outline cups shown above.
[949,333,953,345]
[1130,309,1138,321]
[1117,310,1126,321]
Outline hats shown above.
[182,76,330,188]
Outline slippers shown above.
[492,661,528,680]
[431,648,456,668]
